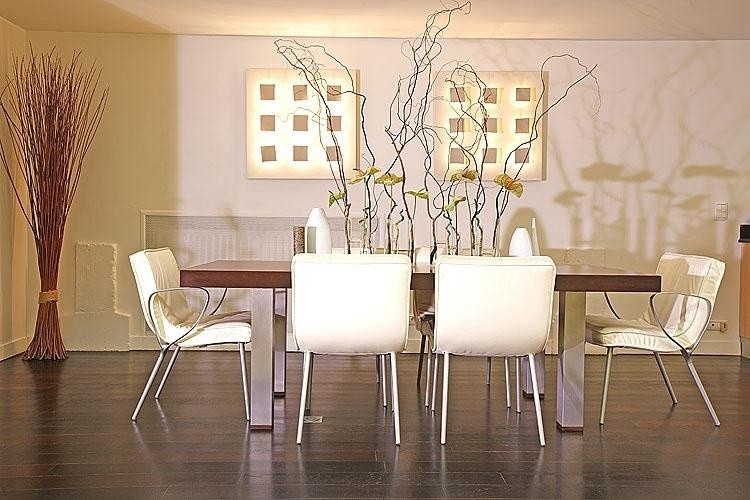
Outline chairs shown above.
[286,250,415,449]
[432,254,561,446]
[585,252,732,430]
[127,243,259,427]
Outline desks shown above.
[174,258,667,438]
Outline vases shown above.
[509,228,533,258]
[304,207,332,256]
